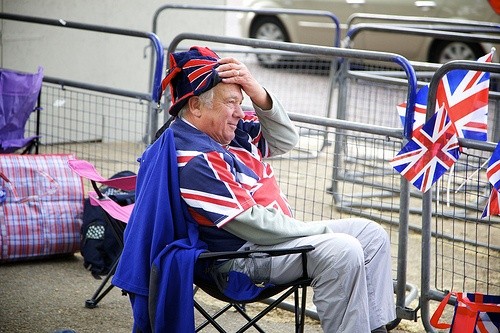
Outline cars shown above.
[242,1,500,81]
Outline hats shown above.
[159,47,226,115]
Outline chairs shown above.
[66,157,139,309]
[187,238,314,333]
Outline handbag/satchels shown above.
[0,154,84,264]
[432,291,500,333]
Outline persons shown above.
[150,46,396,332]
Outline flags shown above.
[390,106,461,193]
[479,140,500,217]
[397,53,492,145]
